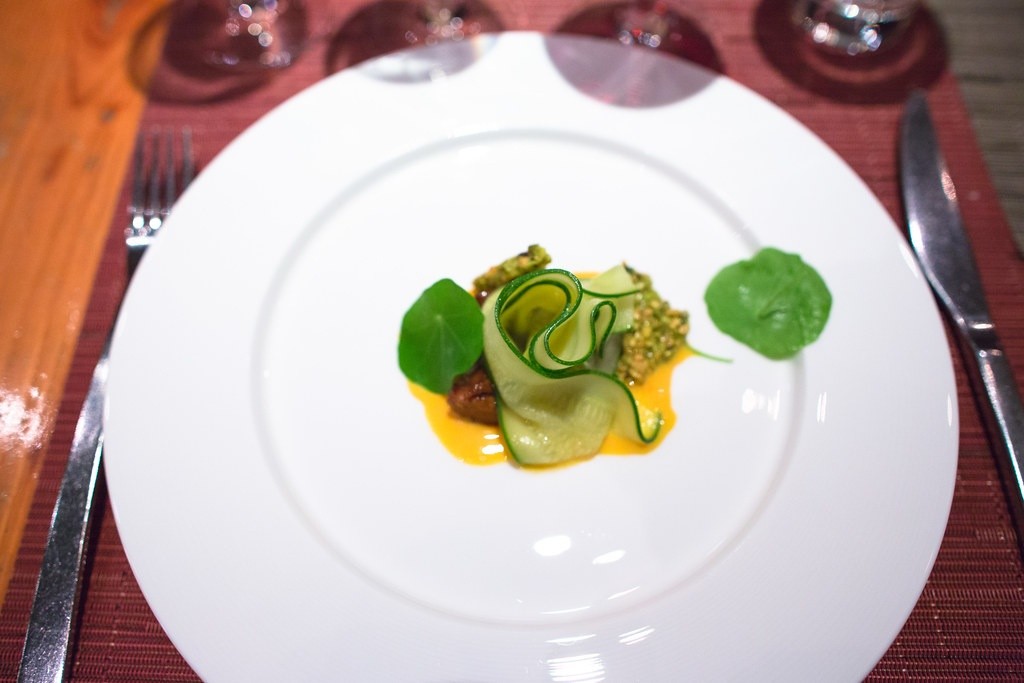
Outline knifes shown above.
[900,96,1024,550]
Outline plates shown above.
[104,31,959,683]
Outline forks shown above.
[16,127,192,682]
